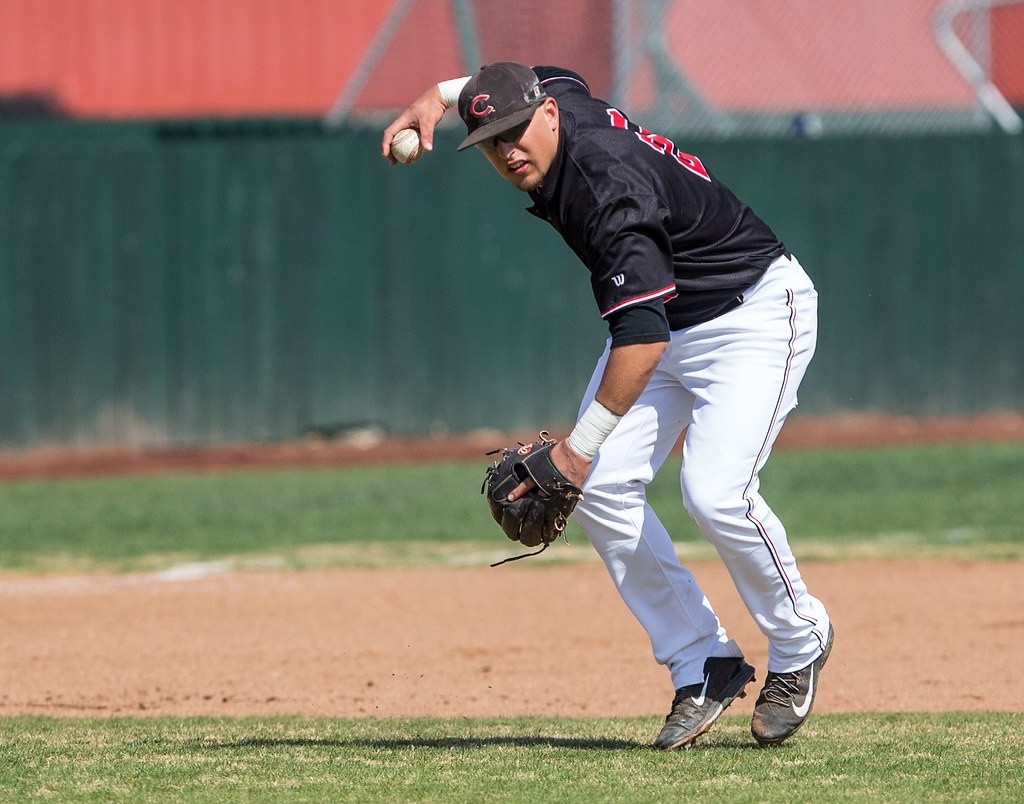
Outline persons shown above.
[381,61,833,750]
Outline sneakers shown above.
[752,621,834,743]
[654,658,757,749]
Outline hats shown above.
[456,62,548,152]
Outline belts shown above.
[666,294,743,330]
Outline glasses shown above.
[475,119,531,152]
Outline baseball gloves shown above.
[487,438,584,547]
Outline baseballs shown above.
[391,128,423,165]
